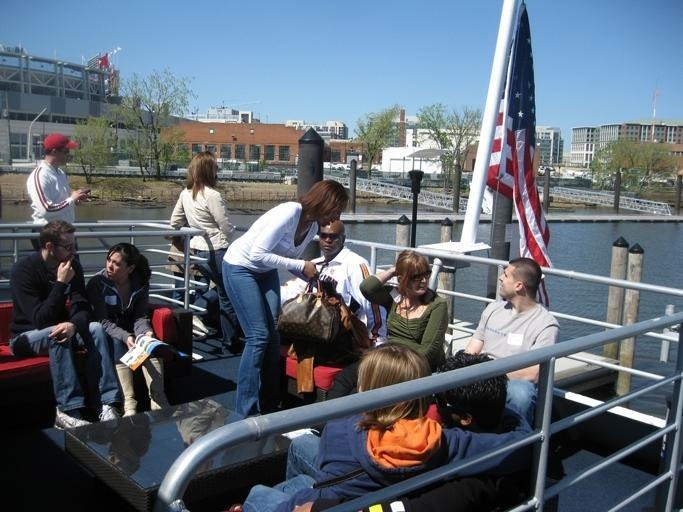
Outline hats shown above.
[44,134,78,150]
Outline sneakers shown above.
[101,405,119,429]
[54,407,92,431]
[192,314,208,336]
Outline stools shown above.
[277,346,347,405]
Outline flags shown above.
[486,1,553,308]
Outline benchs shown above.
[557,450,658,512]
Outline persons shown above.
[171,153,242,354]
[281,221,386,347]
[8,219,123,431]
[169,219,210,300]
[85,242,171,417]
[173,399,229,468]
[466,257,559,424]
[313,249,449,435]
[106,414,153,476]
[274,352,538,512]
[25,132,88,260]
[232,343,534,512]
[222,180,349,420]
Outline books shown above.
[118,333,169,372]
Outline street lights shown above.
[26,106,48,160]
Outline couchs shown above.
[1,297,193,431]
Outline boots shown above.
[142,357,174,410]
[116,364,137,418]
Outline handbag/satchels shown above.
[277,286,368,368]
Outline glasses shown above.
[319,230,341,241]
[408,270,432,283]
[55,243,75,252]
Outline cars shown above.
[536,166,674,187]
[321,160,382,173]
